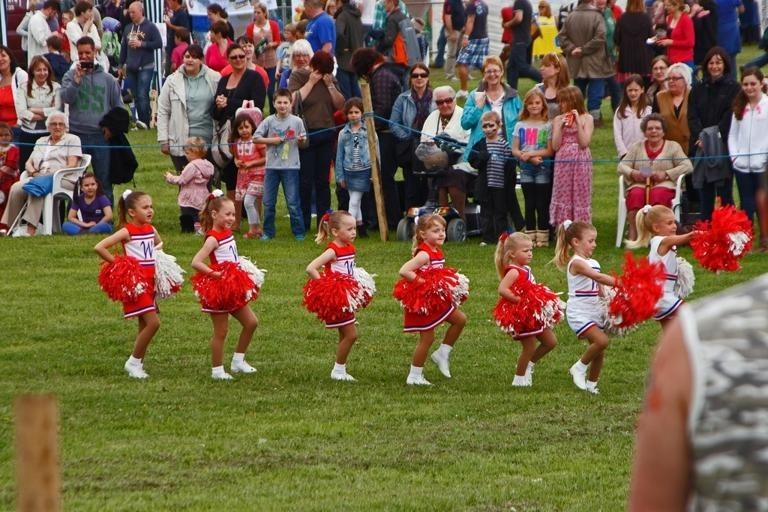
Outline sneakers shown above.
[124,361,146,378]
[241,229,312,242]
[512,379,532,387]
[330,370,358,381]
[431,350,451,378]
[230,360,257,373]
[585,383,599,394]
[570,364,586,390]
[129,372,150,378]
[407,374,431,385]
[515,363,532,385]
[212,372,233,379]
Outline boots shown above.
[526,229,551,248]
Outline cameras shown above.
[80,62,94,69]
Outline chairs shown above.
[615,170,683,249]
[6,153,91,236]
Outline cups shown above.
[565,112,575,127]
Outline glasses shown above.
[435,96,453,106]
[410,73,429,79]
[229,53,246,60]
[664,76,685,81]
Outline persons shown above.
[549,219,626,396]
[93,190,169,378]
[394,212,468,384]
[495,231,558,386]
[193,191,259,381]
[625,172,768,512]
[628,204,713,328]
[304,209,372,384]
